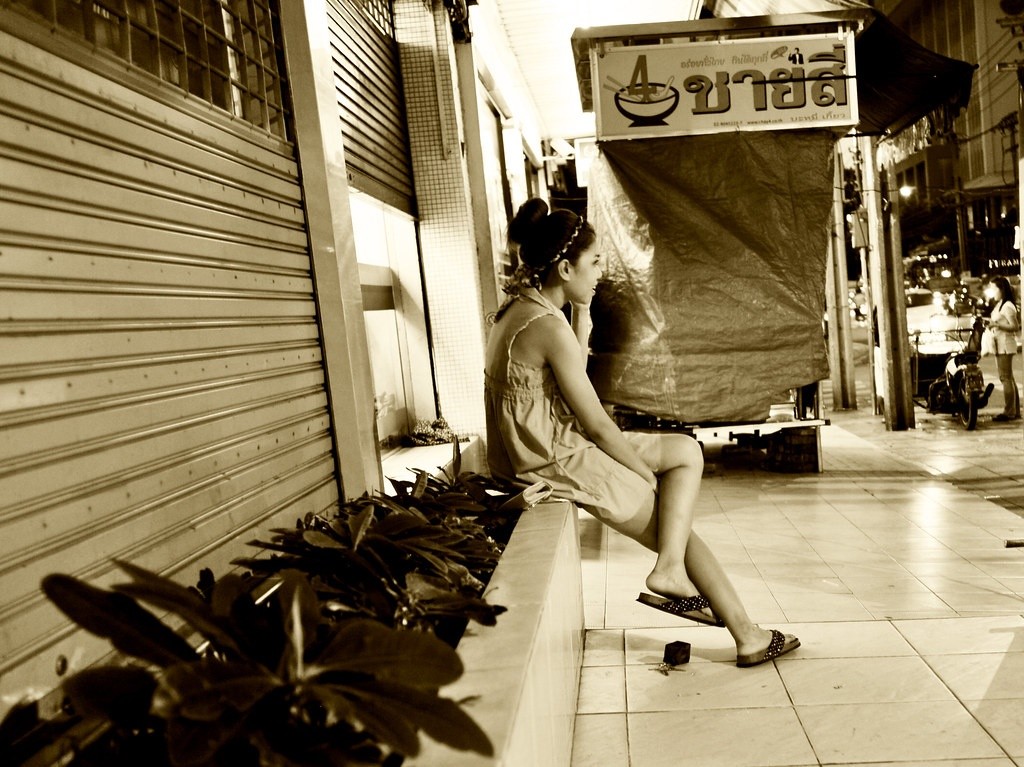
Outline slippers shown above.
[736,629,801,667]
[636,592,725,628]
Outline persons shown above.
[483,199,801,666]
[981,277,1022,422]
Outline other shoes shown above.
[993,414,1021,422]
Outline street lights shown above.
[900,176,967,271]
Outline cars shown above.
[903,280,933,306]
[910,255,969,295]
[943,294,986,318]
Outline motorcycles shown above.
[904,314,994,430]
